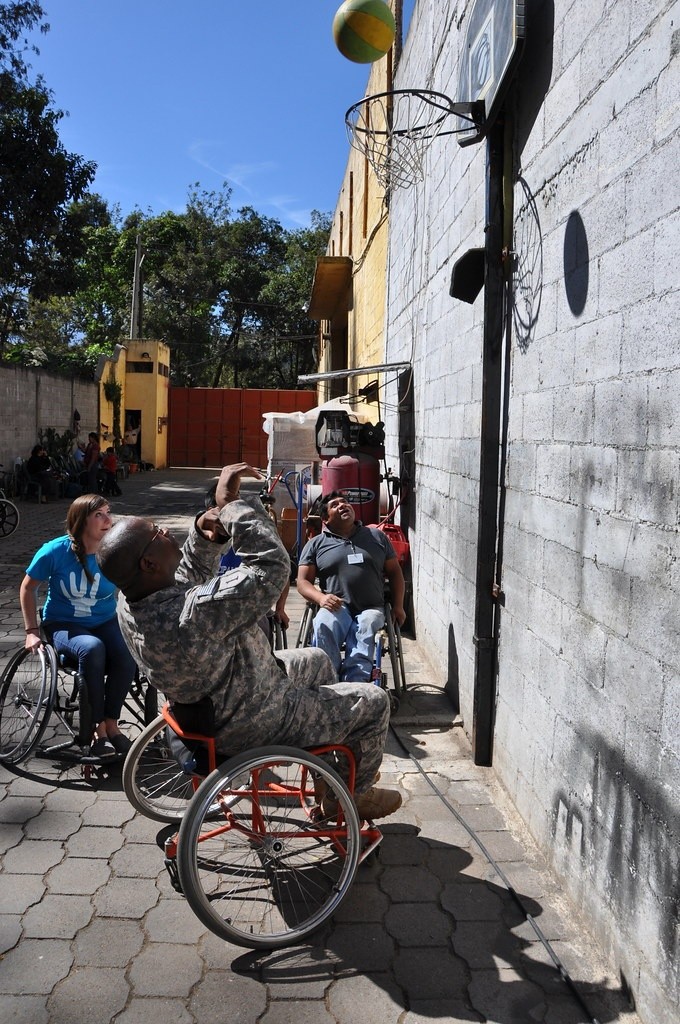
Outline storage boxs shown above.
[271,413,368,462]
[267,463,324,542]
[280,508,307,553]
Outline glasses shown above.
[135,520,164,565]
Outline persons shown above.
[123,424,144,472]
[25,444,83,504]
[73,432,123,497]
[19,493,136,757]
[117,439,139,472]
[296,490,407,683]
[96,461,403,820]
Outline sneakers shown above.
[320,785,401,822]
[311,770,380,804]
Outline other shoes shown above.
[109,733,134,756]
[91,736,116,757]
[41,499,48,504]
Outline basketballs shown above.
[332,0,397,65]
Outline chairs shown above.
[21,462,43,504]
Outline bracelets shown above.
[25,627,40,631]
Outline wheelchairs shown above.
[267,613,287,651]
[295,602,407,715]
[0,464,20,539]
[0,642,169,783]
[124,700,382,952]
